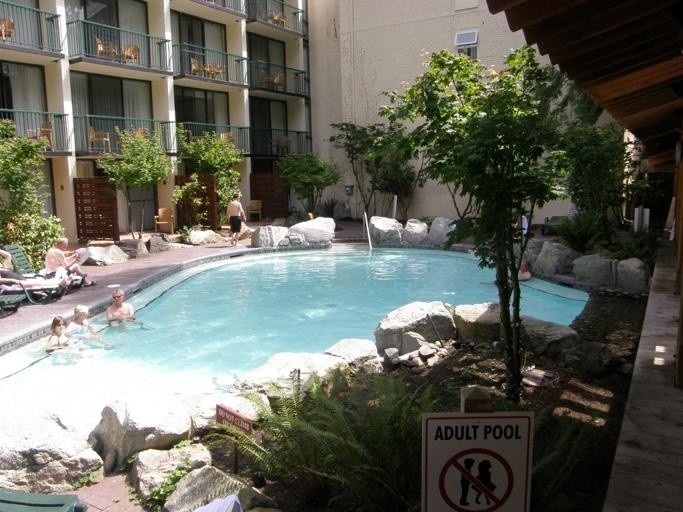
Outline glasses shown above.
[113,296,121,298]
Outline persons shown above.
[0,238,96,288]
[106,288,134,326]
[48,305,93,347]
[227,193,246,246]
[512,206,528,273]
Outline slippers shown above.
[77,272,87,277]
[85,280,97,286]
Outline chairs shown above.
[154,207,175,233]
[26,121,55,151]
[0,17,15,41]
[86,11,292,156]
[0,244,87,316]
[247,200,262,222]
